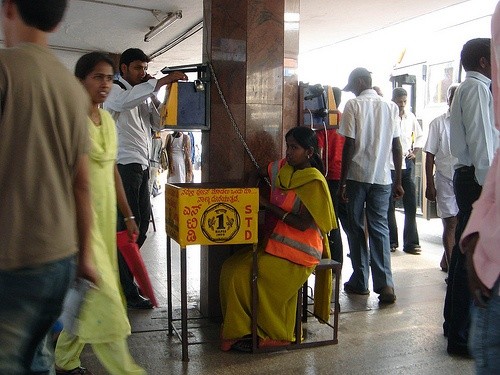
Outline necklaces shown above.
[90,113,101,126]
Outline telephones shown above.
[315,83,328,118]
[143,73,148,82]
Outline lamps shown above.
[144,12,183,43]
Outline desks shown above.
[164,180,260,361]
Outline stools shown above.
[293,258,342,349]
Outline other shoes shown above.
[258,338,291,348]
[126,295,154,309]
[220,337,241,351]
[403,245,421,255]
[390,247,396,252]
[344,281,370,295]
[54,363,93,375]
[377,288,396,306]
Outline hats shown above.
[342,67,372,92]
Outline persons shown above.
[423,83,460,285]
[315,87,348,272]
[387,88,422,254]
[460,0,500,375]
[102,48,188,308]
[218,127,337,351]
[54,52,148,375]
[166,131,192,184]
[0,0,101,375]
[336,68,404,303]
[443,38,500,356]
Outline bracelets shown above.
[124,216,135,221]
[282,213,288,220]
